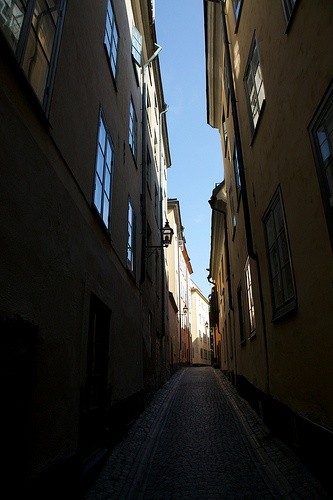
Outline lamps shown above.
[143,220,174,257]
[176,304,188,315]
[205,322,210,328]
